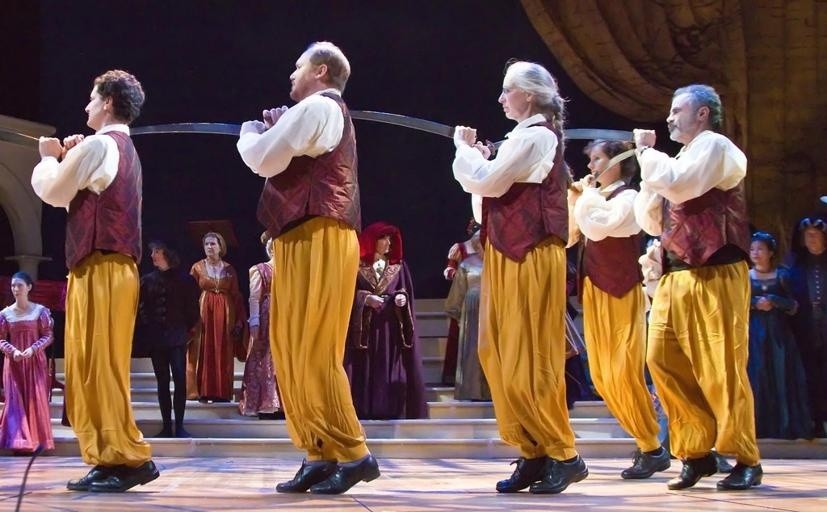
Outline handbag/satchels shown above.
[636,145,652,156]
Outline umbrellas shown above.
[277,459,336,492]
[668,457,718,489]
[622,446,671,479]
[68,465,103,491]
[307,454,380,495]
[718,463,763,489]
[529,454,589,494]
[496,456,552,492]
[91,461,160,492]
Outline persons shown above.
[30,69,160,493]
[746,230,814,441]
[453,61,589,494]
[235,230,286,420]
[566,259,588,410]
[706,442,735,473]
[564,137,671,478]
[782,222,805,268]
[189,231,250,403]
[235,40,381,495]
[442,218,477,387]
[345,220,429,419]
[0,272,56,455]
[138,234,197,438]
[442,228,493,402]
[783,218,824,437]
[633,86,763,491]
